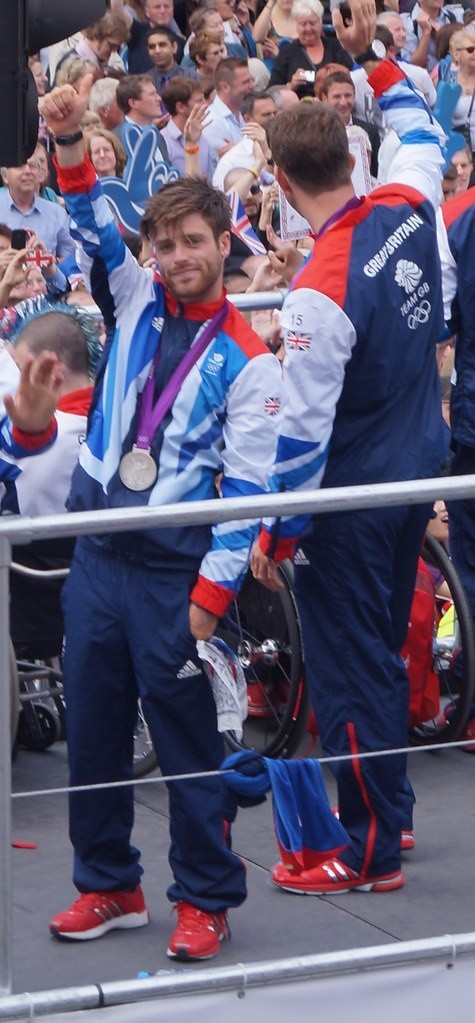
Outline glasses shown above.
[456,47,474,54]
[250,184,261,195]
[104,38,120,50]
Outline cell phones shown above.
[299,71,315,83]
[11,229,26,251]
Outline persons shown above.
[250,0,450,896]
[37,74,284,962]
[1,0,475,749]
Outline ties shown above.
[160,76,171,117]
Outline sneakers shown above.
[326,806,415,849]
[270,852,405,895]
[49,883,151,942]
[167,897,232,961]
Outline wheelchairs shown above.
[11,501,473,770]
